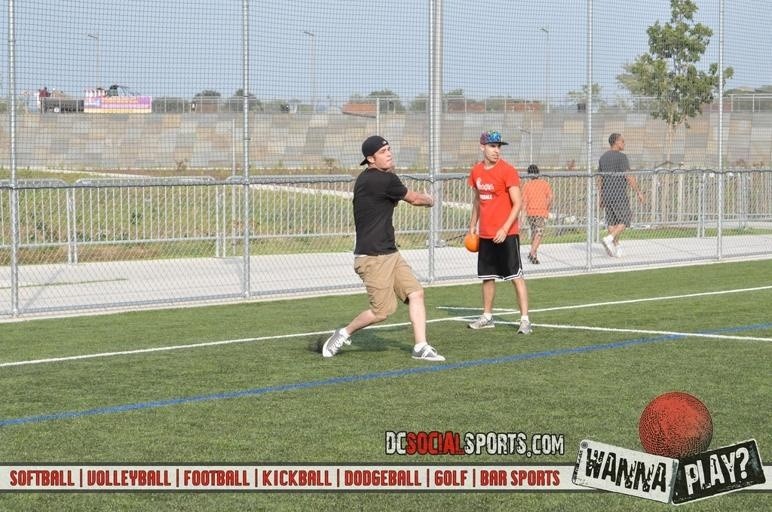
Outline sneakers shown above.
[323,327,350,358]
[468,316,495,329]
[616,246,624,258]
[412,344,446,361]
[527,253,538,263]
[601,237,615,256]
[516,320,533,334]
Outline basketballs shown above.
[464,233,479,252]
[640,391,713,460]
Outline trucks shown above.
[35,87,82,116]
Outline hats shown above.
[480,130,507,144]
[360,136,388,165]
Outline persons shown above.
[468,130,533,336]
[594,133,645,258]
[20,85,69,114]
[522,166,552,265]
[321,136,446,362]
